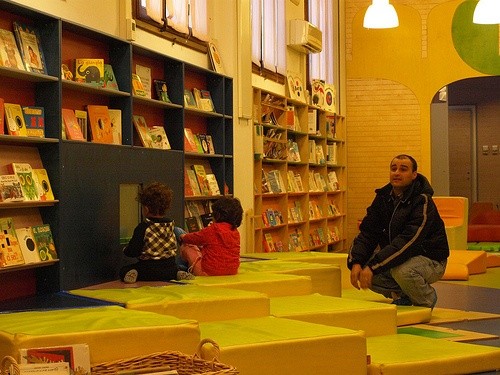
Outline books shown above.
[0,98,45,138]
[0,162,55,201]
[184,165,221,234]
[262,168,342,193]
[9,344,91,375]
[0,20,49,74]
[264,227,342,252]
[132,64,172,149]
[185,87,217,155]
[61,58,123,146]
[261,200,341,228]
[286,106,338,166]
[0,217,58,268]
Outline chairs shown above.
[468,202,500,243]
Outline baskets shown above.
[1,337,240,375]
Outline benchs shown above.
[432,196,469,250]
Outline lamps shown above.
[472,0,500,25]
[362,0,399,29]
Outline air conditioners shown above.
[287,17,322,55]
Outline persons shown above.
[174,197,243,276]
[347,154,450,311]
[118,182,195,283]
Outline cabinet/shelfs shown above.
[0,0,60,302]
[252,86,347,254]
[184,61,234,233]
[60,16,185,292]
